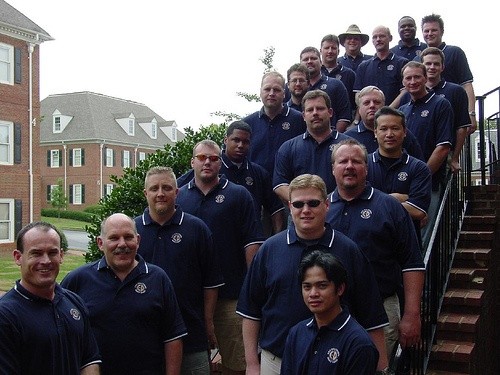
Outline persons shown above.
[283,46,353,133]
[400,46,473,176]
[345,26,410,131]
[278,249,379,375]
[134,166,227,375]
[389,15,428,60]
[236,70,306,175]
[364,106,431,258]
[383,60,454,222]
[319,33,356,109]
[176,139,268,364]
[411,13,477,134]
[59,213,188,375]
[0,221,102,375]
[235,172,391,375]
[342,85,423,162]
[273,90,368,230]
[174,122,285,236]
[323,136,425,375]
[286,64,311,112]
[338,23,374,72]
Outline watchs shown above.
[377,367,390,375]
[353,119,360,126]
[468,110,476,117]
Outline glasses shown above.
[290,199,322,208]
[193,154,221,162]
[231,137,250,144]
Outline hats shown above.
[338,24,369,47]
